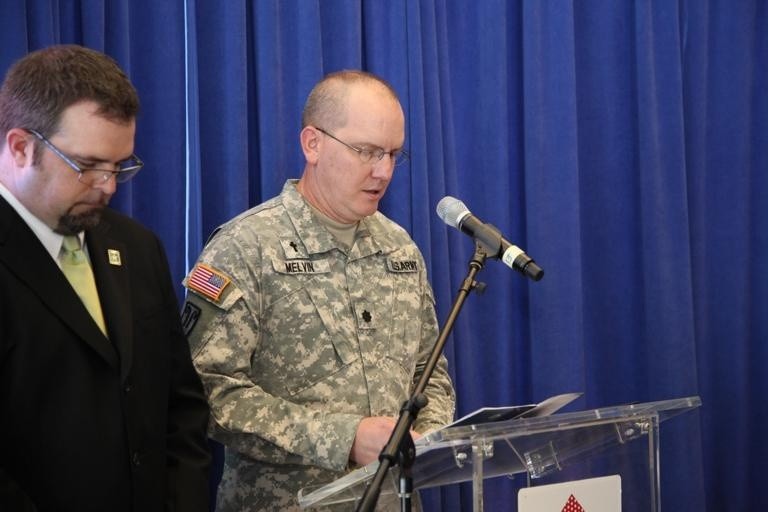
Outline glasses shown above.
[28,132,144,187]
[318,127,412,168]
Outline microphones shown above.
[436,195,544,282]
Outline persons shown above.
[178,70,458,512]
[0,44,217,509]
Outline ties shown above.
[61,232,107,338]
